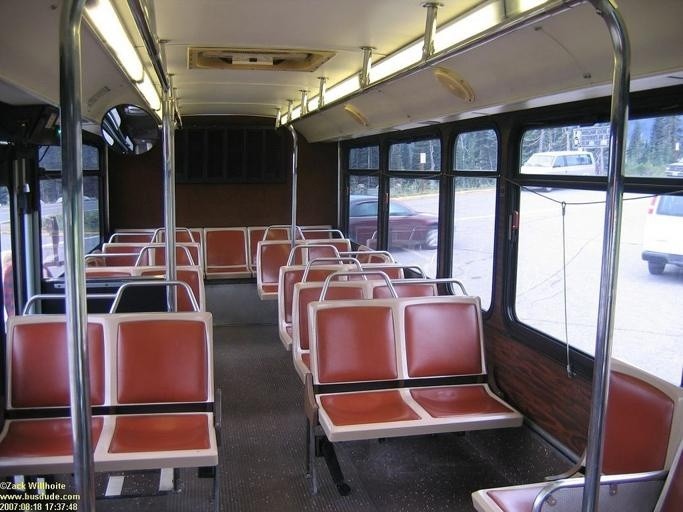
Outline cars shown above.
[56,195,89,203]
[348,195,438,251]
[665,162,683,178]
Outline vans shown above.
[640,194,683,275]
[520,150,598,193]
